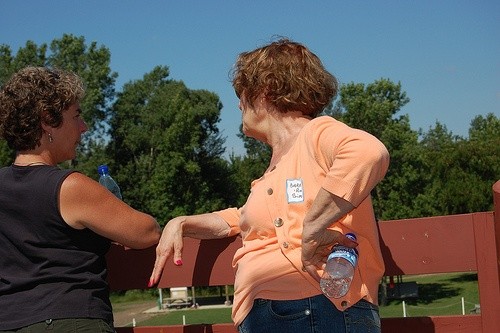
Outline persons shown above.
[1,66,163,332]
[148,35,390,333]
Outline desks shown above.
[163,297,192,309]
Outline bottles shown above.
[98,163,122,202]
[318,232,360,299]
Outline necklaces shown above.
[12,162,48,166]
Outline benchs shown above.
[165,303,191,306]
